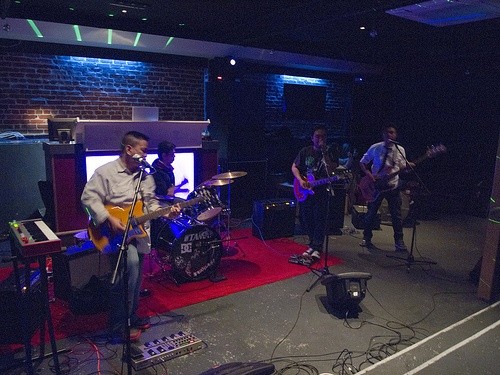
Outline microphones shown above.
[387,139,399,144]
[318,141,325,156]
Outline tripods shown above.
[214,183,239,253]
[305,157,334,292]
[385,144,437,272]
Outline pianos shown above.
[0,217,72,375]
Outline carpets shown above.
[0,227,342,352]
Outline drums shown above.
[187,188,223,220]
[155,215,222,281]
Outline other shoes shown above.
[131,319,150,329]
[359,239,371,245]
[109,326,142,340]
[311,250,320,259]
[395,240,407,249]
[303,247,314,256]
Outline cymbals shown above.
[203,180,234,186]
[212,171,247,179]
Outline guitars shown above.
[294,170,351,202]
[88,189,212,254]
[360,143,447,202]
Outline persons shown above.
[150,140,176,197]
[79,131,181,342]
[358,121,416,251]
[291,127,339,261]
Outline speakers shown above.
[0,287,38,344]
[320,271,372,307]
[252,198,296,239]
[52,240,114,298]
[300,189,346,234]
[352,205,381,230]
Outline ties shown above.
[377,145,389,174]
